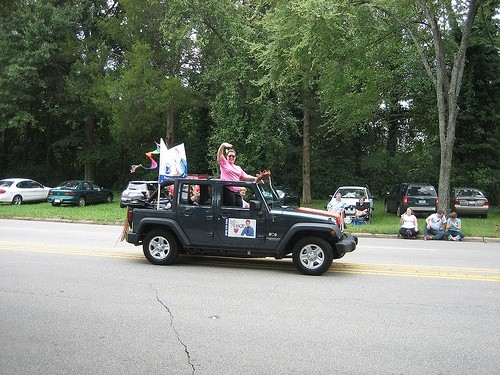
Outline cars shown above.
[327,185,374,217]
[449,188,488,219]
[47,180,114,207]
[254,185,301,208]
[120,181,168,208]
[1,178,54,205]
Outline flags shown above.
[158,139,187,183]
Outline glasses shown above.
[228,155,235,157]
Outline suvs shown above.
[169,173,214,202]
[125,178,359,277]
[383,182,438,216]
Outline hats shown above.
[449,212,457,218]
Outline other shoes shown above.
[403,236,464,241]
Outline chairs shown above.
[356,202,371,224]
[232,193,243,208]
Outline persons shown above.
[423,210,446,240]
[326,193,347,213]
[353,198,369,226]
[241,220,254,236]
[400,208,419,238]
[217,143,256,210]
[181,186,196,206]
[446,212,465,241]
